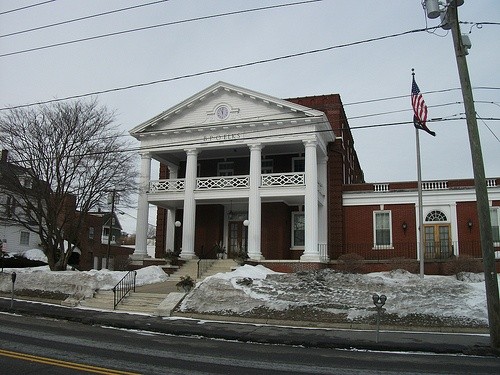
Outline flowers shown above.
[213,240,226,257]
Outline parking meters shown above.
[372,293,387,342]
[10,272,17,308]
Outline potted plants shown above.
[162,249,176,265]
[176,274,194,293]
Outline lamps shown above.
[468,219,473,231]
[228,200,233,219]
[243,220,249,227]
[402,222,406,233]
[174,221,181,227]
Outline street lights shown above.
[175,220,181,254]
[243,220,248,255]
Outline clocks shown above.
[215,106,229,120]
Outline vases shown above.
[217,253,223,259]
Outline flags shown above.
[411,77,436,137]
